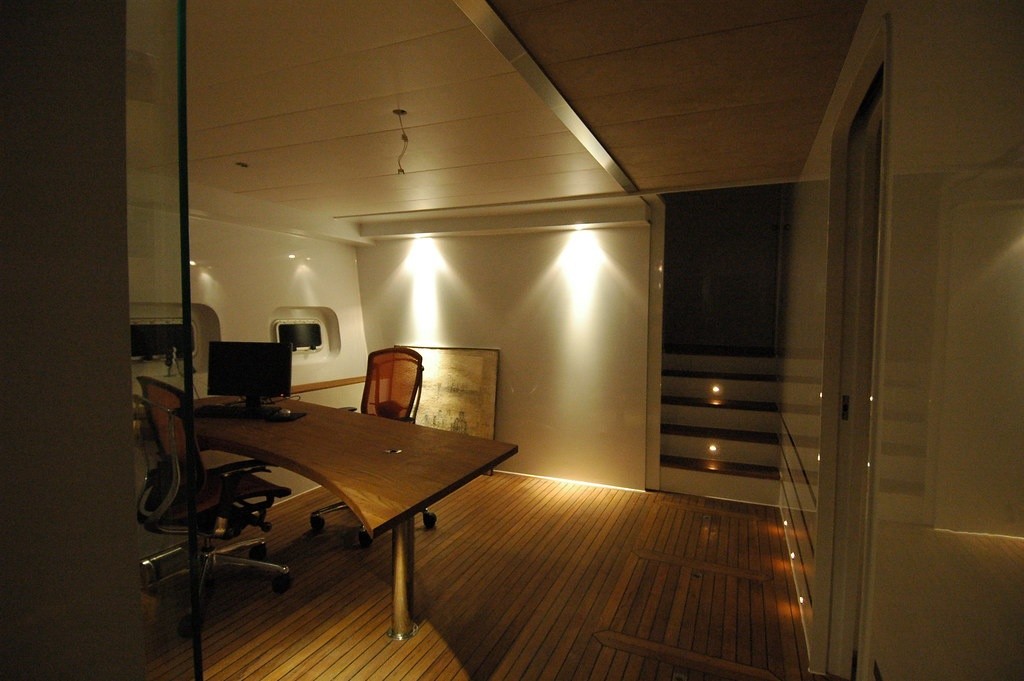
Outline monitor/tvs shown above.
[208,342,292,410]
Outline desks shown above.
[130,396,518,642]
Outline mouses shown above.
[279,409,291,417]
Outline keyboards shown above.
[196,405,281,420]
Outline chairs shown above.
[310,348,438,547]
[135,377,290,639]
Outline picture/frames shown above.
[395,345,501,476]
